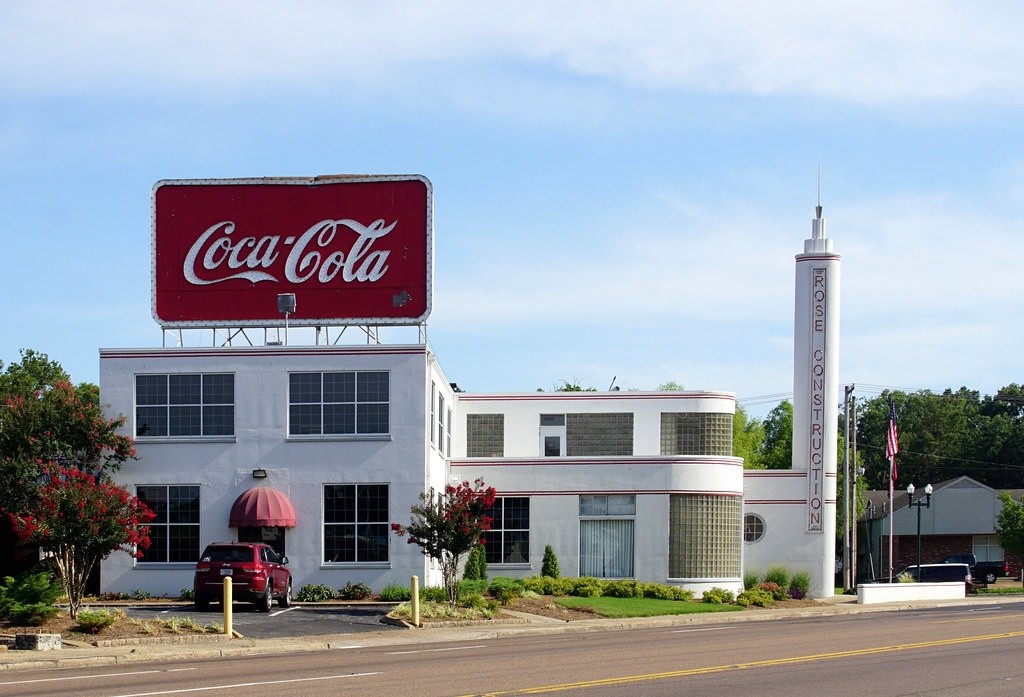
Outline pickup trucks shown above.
[937,552,1009,584]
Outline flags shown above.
[885,399,899,481]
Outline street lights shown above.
[907,483,933,582]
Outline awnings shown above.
[228,485,298,528]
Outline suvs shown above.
[193,541,293,614]
[873,563,975,596]
